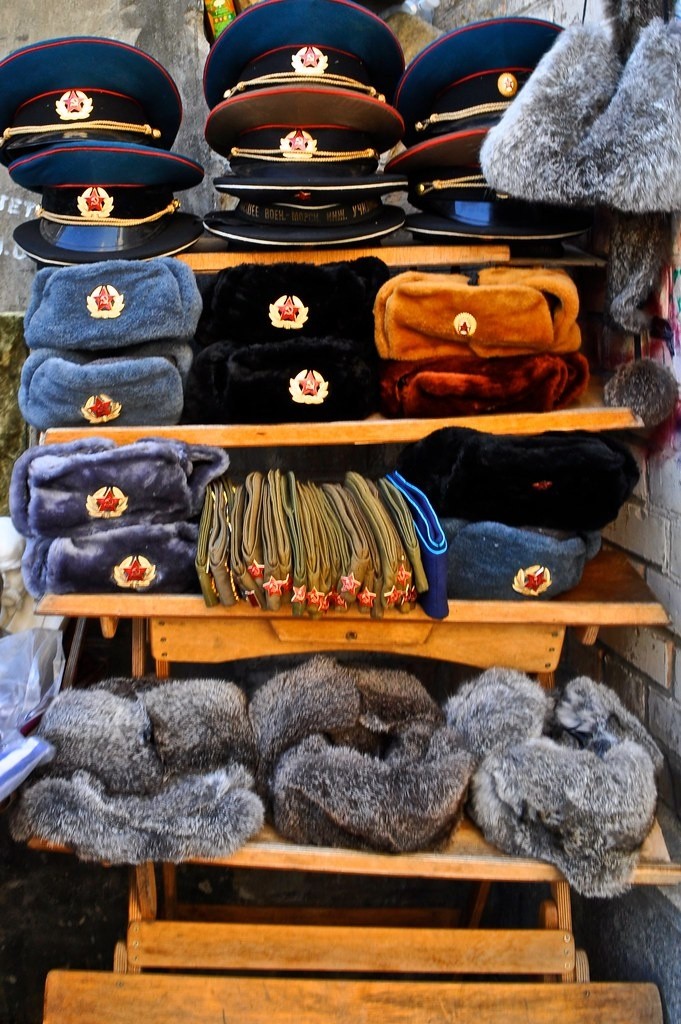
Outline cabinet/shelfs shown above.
[25,234,681,1024]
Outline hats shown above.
[0,0,681,425]
[9,426,640,618]
[7,657,664,897]
